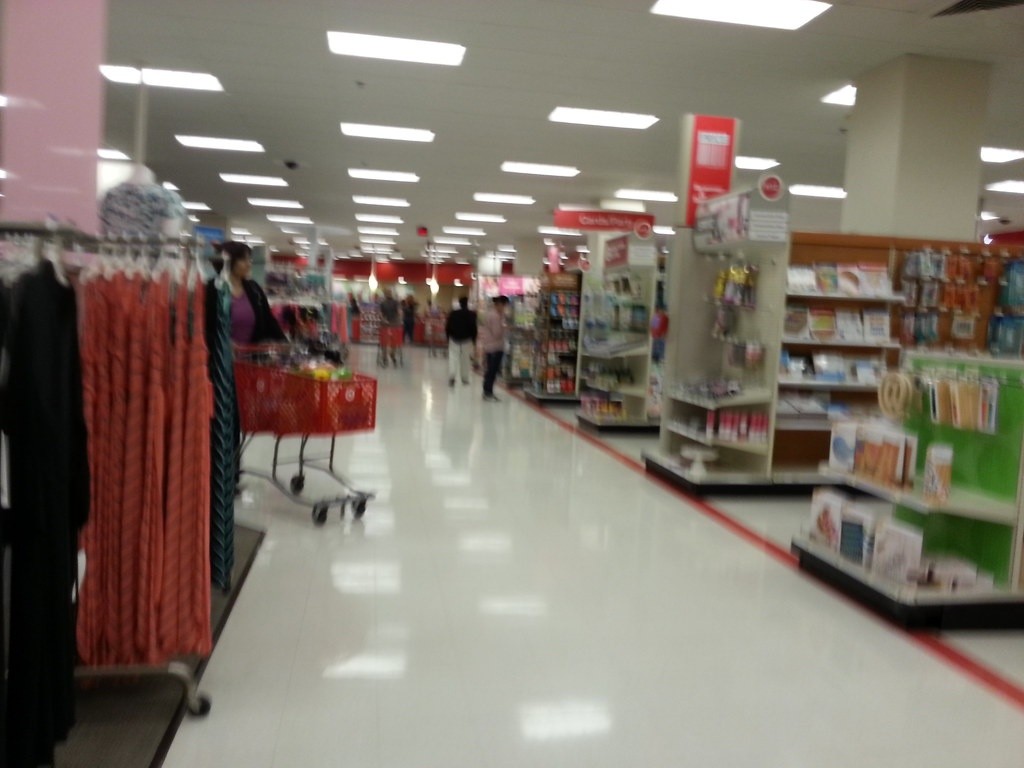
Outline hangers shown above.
[0,217,236,294]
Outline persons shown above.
[401,294,419,343]
[87,158,182,240]
[203,241,292,357]
[482,291,512,403]
[345,288,379,312]
[421,299,441,356]
[444,295,478,386]
[376,288,400,366]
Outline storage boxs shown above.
[707,409,771,444]
[580,391,627,418]
[815,486,923,590]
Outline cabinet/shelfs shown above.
[497,273,584,407]
[641,228,1024,498]
[575,265,666,433]
[792,350,1024,632]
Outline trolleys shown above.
[379,322,404,370]
[226,333,378,527]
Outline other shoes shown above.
[450,379,455,387]
[483,395,501,402]
[462,381,469,384]
